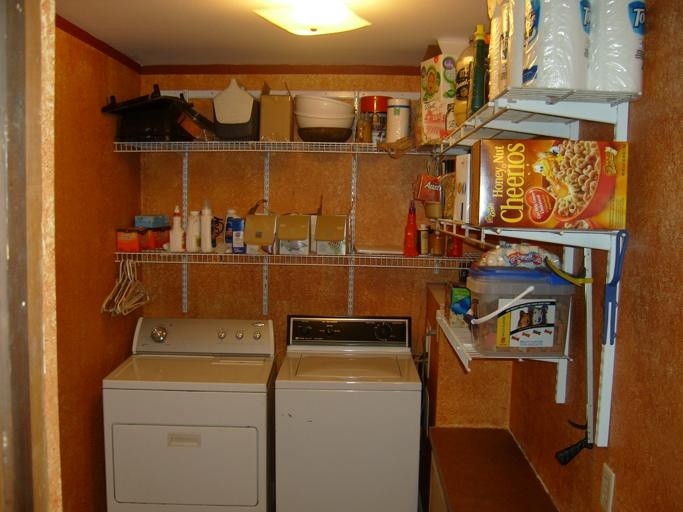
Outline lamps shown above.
[253,1,371,37]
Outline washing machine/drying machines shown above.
[275,315,422,512]
[99,315,277,512]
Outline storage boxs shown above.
[465,265,575,358]
[259,81,294,143]
[419,39,471,144]
[245,214,350,257]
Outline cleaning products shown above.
[199,201,216,252]
[403,200,418,257]
[168,205,185,253]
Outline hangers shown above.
[99,259,149,317]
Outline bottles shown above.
[403,200,443,258]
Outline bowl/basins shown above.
[294,94,356,142]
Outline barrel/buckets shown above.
[184,210,200,253]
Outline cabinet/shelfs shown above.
[110,89,476,317]
[420,283,515,429]
[432,86,641,450]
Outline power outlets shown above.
[599,462,615,512]
